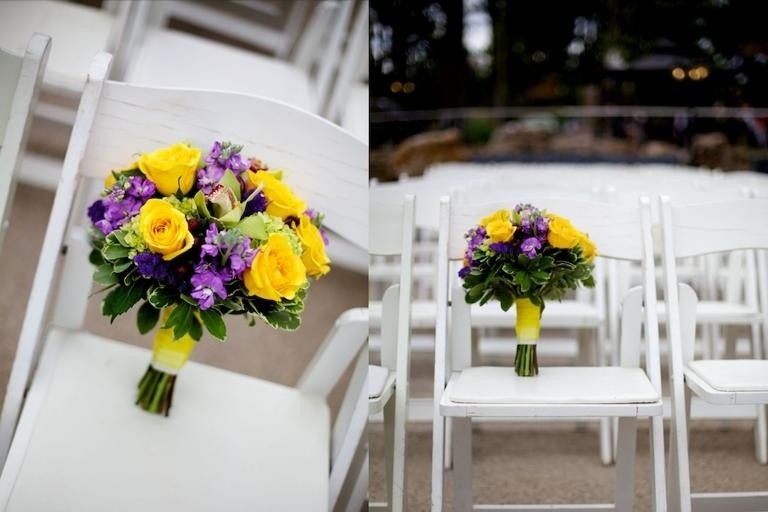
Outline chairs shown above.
[369,163,768,511]
[2,1,369,511]
